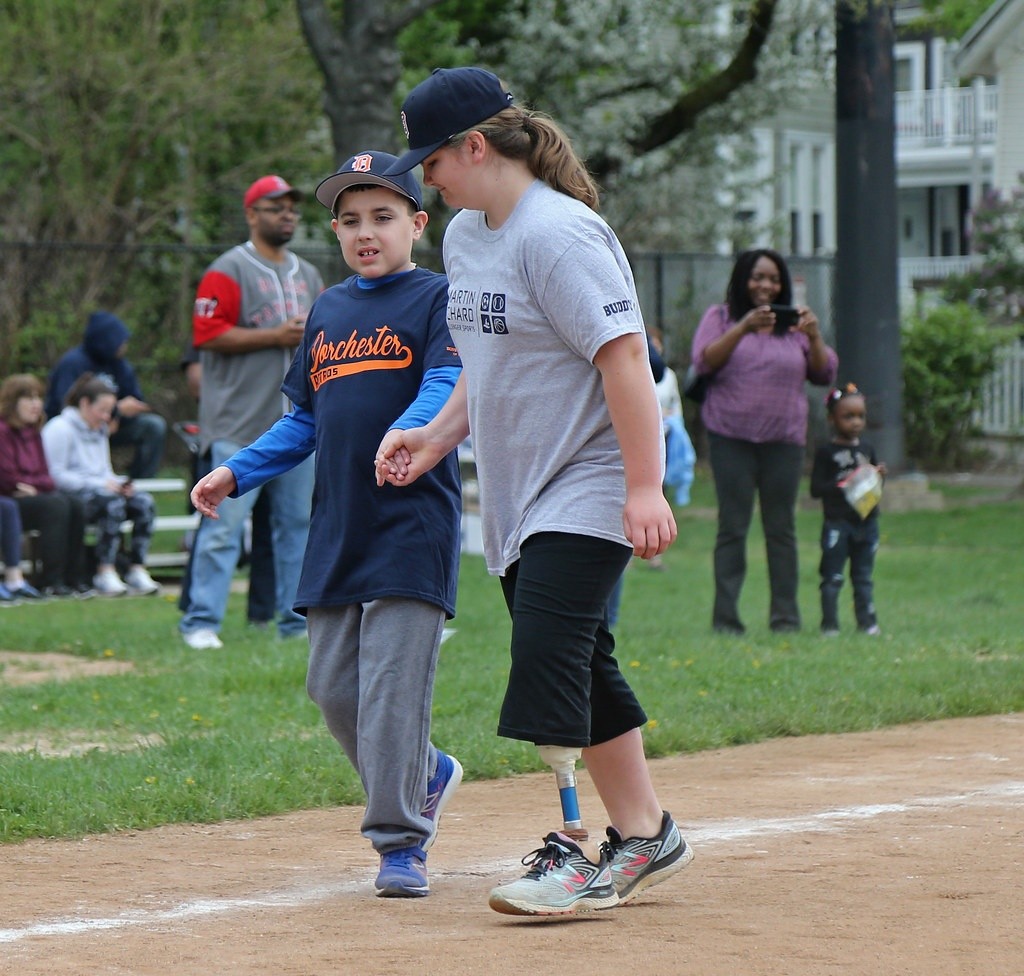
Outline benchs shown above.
[0,477,200,572]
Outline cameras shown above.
[771,306,800,326]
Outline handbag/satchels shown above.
[685,306,729,406]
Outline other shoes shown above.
[180,625,224,648]
[711,614,742,633]
[820,619,841,636]
[45,581,83,602]
[770,617,800,632]
[90,572,129,596]
[4,580,49,606]
[0,582,21,608]
[124,567,162,596]
[70,578,98,600]
[857,623,882,635]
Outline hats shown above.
[245,176,301,206]
[381,67,514,175]
[316,151,423,211]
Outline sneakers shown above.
[602,812,694,906]
[421,748,464,851]
[489,829,620,916]
[375,849,429,897]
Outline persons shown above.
[45,312,167,478]
[810,383,887,637]
[374,67,697,915]
[175,174,328,649]
[191,150,464,898]
[692,249,838,635]
[0,372,160,608]
